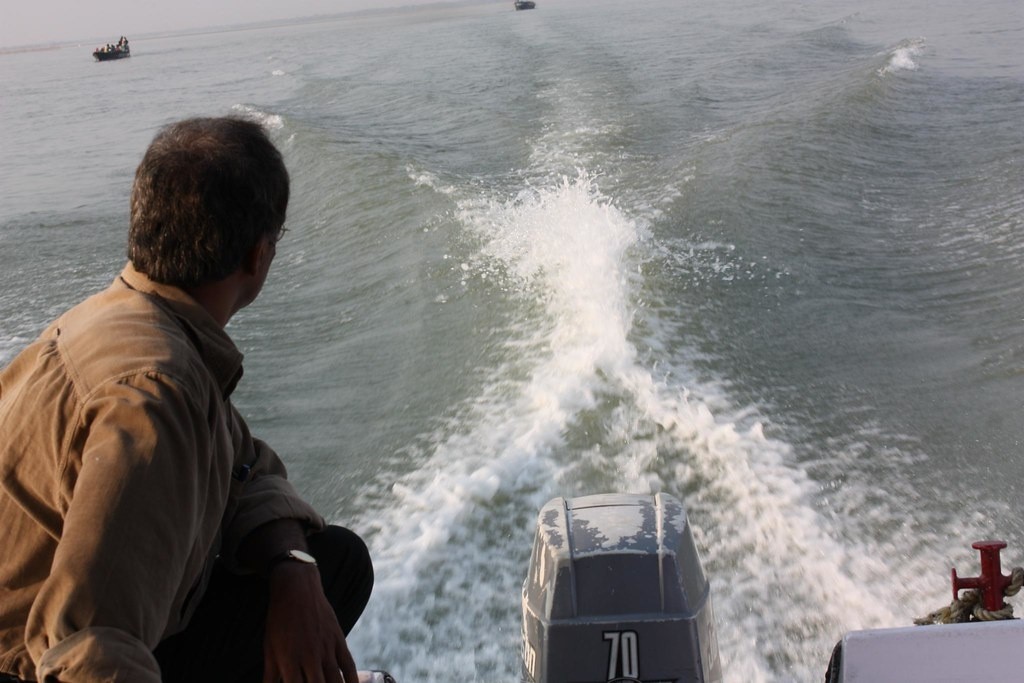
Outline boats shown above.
[514,1,536,11]
[91,36,130,62]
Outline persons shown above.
[96,36,128,53]
[0,117,396,683]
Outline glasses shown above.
[264,222,291,241]
